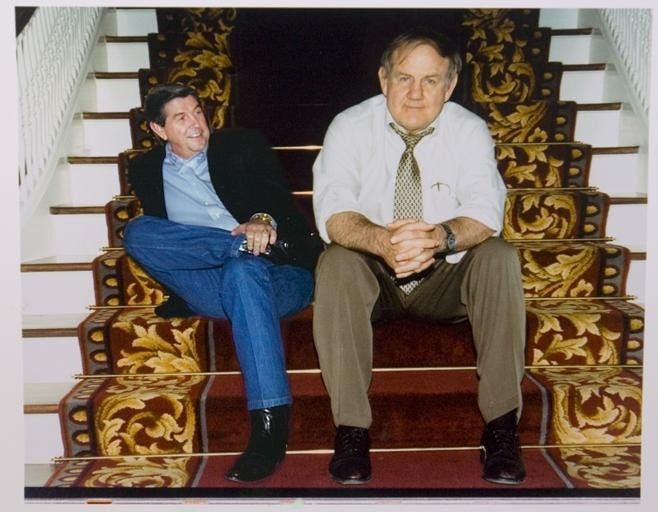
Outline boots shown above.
[226,405,287,484]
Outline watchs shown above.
[249,213,273,225]
[439,222,457,255]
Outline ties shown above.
[390,122,435,295]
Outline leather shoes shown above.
[480,428,526,485]
[329,426,372,484]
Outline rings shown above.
[262,229,268,233]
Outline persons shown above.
[124,82,326,483]
[309,31,528,488]
